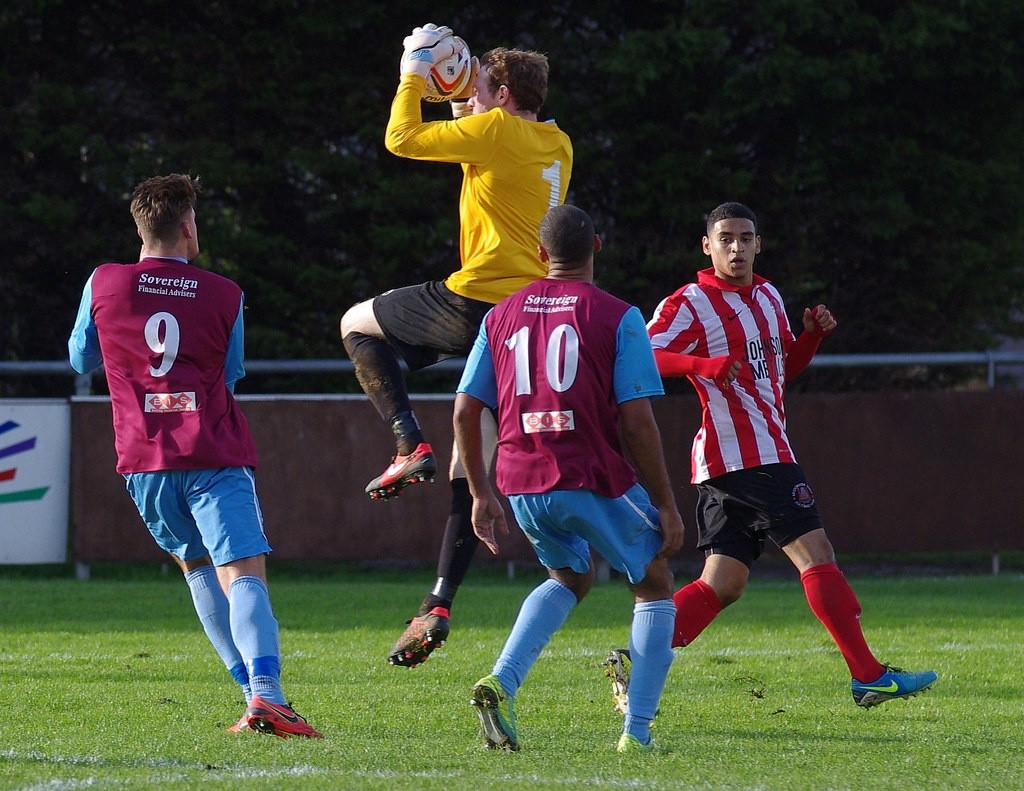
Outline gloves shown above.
[401,24,464,79]
[451,54,481,120]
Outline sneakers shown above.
[850,663,936,711]
[469,673,521,752]
[388,606,450,669]
[247,695,324,742]
[616,733,661,753]
[363,442,437,502]
[226,709,252,733]
[606,647,631,716]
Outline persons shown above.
[340,24,572,668]
[452,204,685,753]
[602,202,936,719]
[67,175,324,737]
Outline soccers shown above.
[400,35,470,103]
